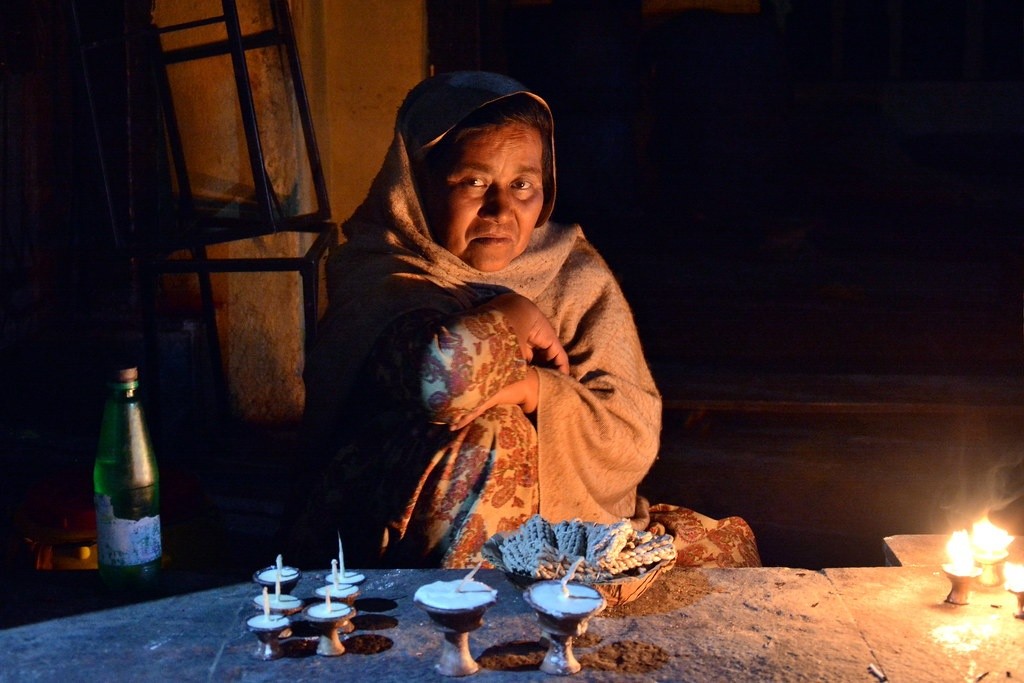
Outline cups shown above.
[244,565,366,660]
[522,580,607,676]
[414,582,496,677]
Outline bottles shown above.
[93,368,159,585]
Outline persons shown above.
[295,71,664,571]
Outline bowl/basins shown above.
[481,522,678,608]
